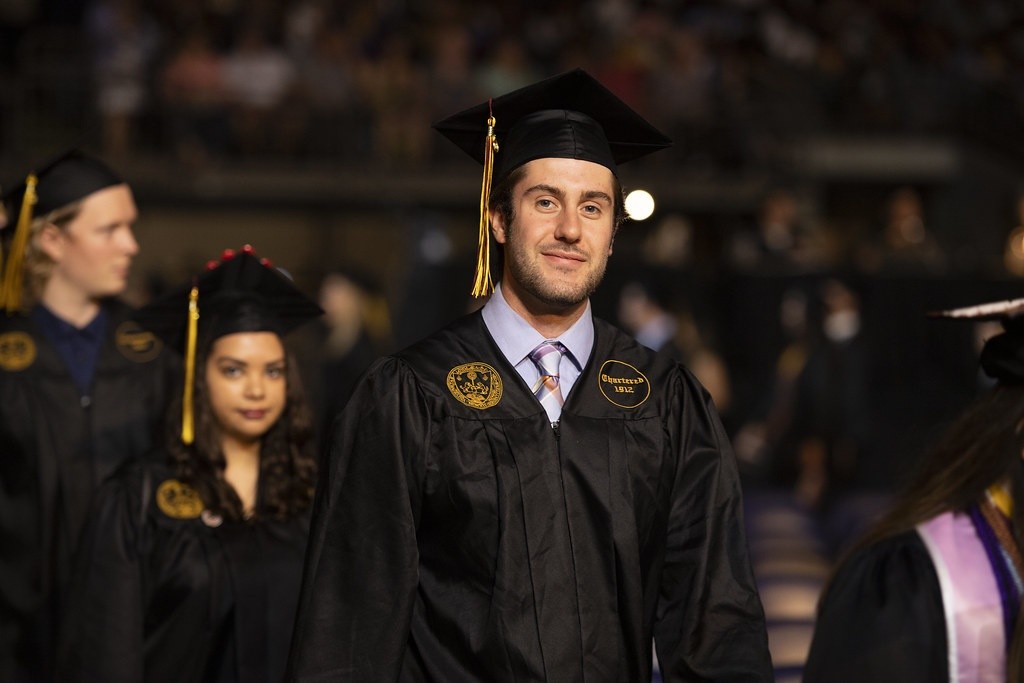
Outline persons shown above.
[0,147,483,683]
[799,295,1024,683]
[293,67,777,683]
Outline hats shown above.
[915,291,1024,397]
[1,119,127,314]
[133,249,329,446]
[431,66,675,303]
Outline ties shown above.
[526,340,570,429]
